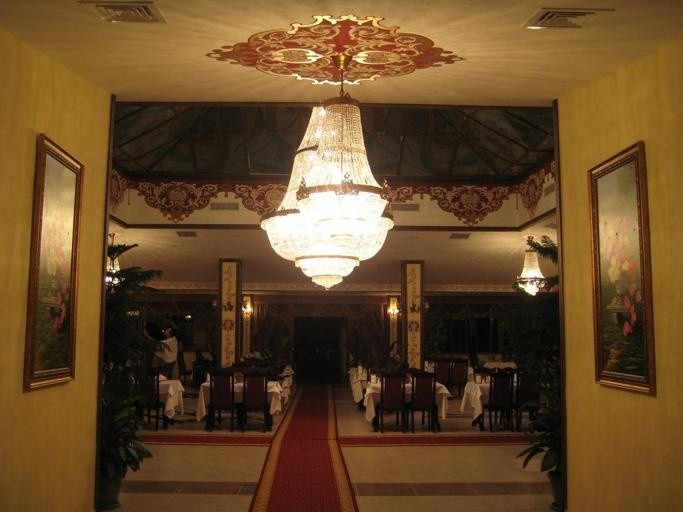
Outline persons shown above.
[142,322,179,380]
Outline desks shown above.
[155,378,184,424]
[279,364,295,387]
[347,364,356,381]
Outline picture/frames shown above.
[22,132,85,394]
[585,139,658,397]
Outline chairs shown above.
[351,357,544,438]
[176,350,284,435]
[133,366,166,431]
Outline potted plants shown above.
[520,341,568,509]
[101,265,168,509]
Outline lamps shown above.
[256,50,394,296]
[517,235,545,297]
